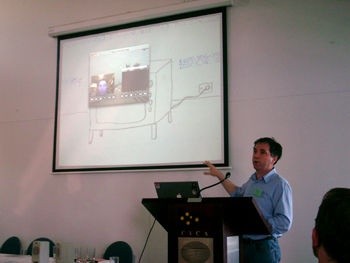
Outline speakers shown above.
[32,241,50,263]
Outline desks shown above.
[0,252,56,263]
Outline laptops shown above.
[154,181,201,199]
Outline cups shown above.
[109,257,119,263]
[75,246,96,263]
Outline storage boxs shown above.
[32,241,49,263]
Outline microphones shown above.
[200,172,232,192]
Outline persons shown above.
[202,137,293,263]
[312,187,350,263]
[98,80,108,97]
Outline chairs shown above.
[102,238,135,263]
[24,237,56,256]
[0,236,23,254]
